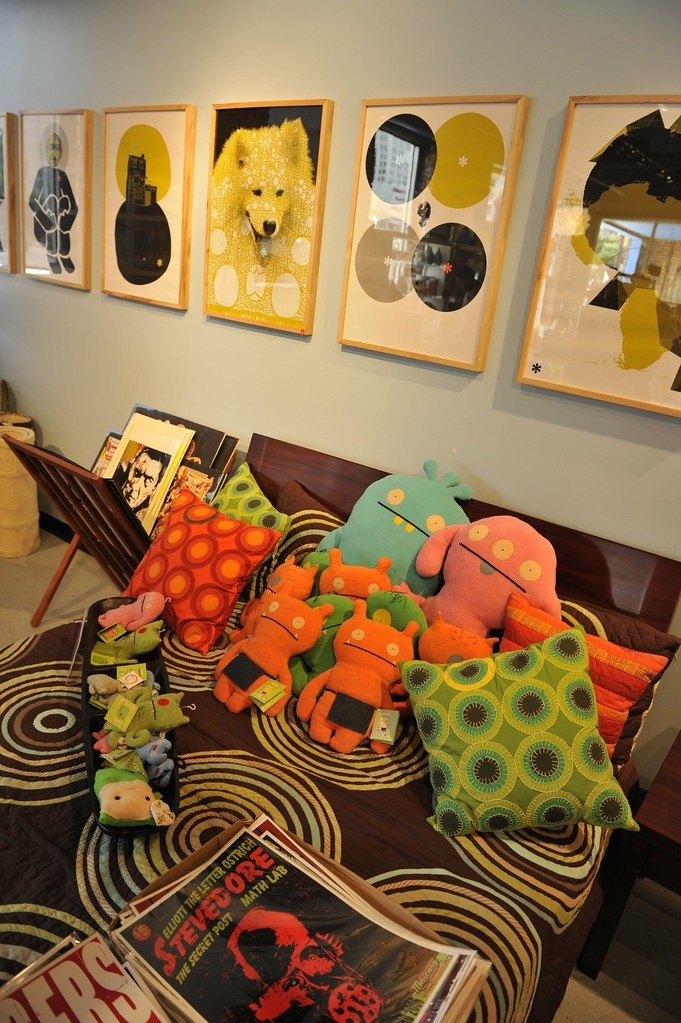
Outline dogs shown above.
[205,114,316,322]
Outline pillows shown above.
[122,459,293,656]
[398,593,670,837]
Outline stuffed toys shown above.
[296,599,421,755]
[76,456,564,827]
[214,592,335,715]
[418,609,499,666]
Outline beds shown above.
[0,433,681,1023]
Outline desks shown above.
[574,731,681,981]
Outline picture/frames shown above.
[203,99,335,336]
[517,94,681,419]
[1,112,16,274]
[338,96,532,371]
[102,104,198,311]
[15,108,96,289]
